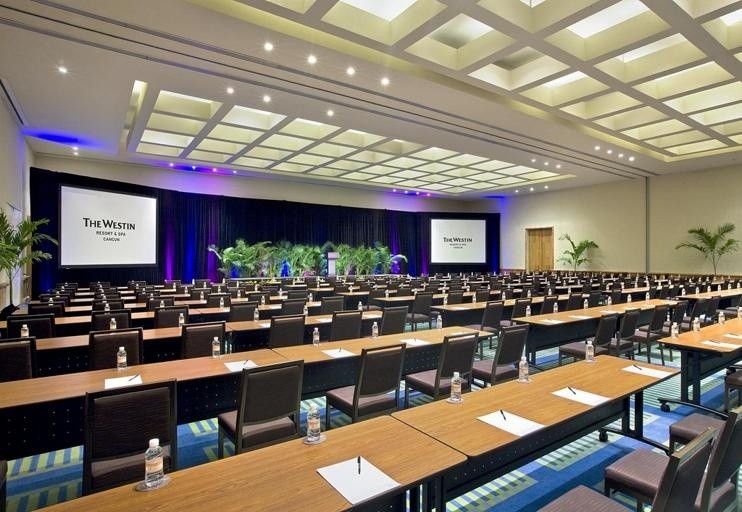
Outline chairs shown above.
[0,271,742,512]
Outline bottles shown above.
[102,295,107,304]
[303,305,309,318]
[20,324,29,346]
[371,321,379,338]
[357,300,363,311]
[211,336,220,358]
[254,308,259,323]
[279,288,282,297]
[98,281,105,296]
[346,268,740,305]
[179,312,185,329]
[237,289,241,300]
[317,281,320,287]
[61,282,68,291]
[56,291,60,298]
[220,298,225,309]
[143,438,166,490]
[718,311,725,326]
[261,295,265,307]
[309,293,313,303]
[670,323,679,338]
[307,404,321,442]
[117,347,128,372]
[437,314,443,331]
[200,291,204,301]
[48,298,53,306]
[519,356,530,381]
[132,280,155,300]
[525,306,531,318]
[692,317,701,334]
[105,303,110,316]
[313,327,320,347]
[110,317,117,335]
[585,341,594,360]
[553,302,558,314]
[159,300,166,311]
[164,278,297,295]
[738,306,742,321]
[450,371,463,402]
[583,299,588,310]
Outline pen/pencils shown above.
[500,409,506,420]
[244,359,248,365]
[568,386,576,394]
[358,456,361,474]
[633,365,642,370]
[339,347,341,352]
[129,373,139,381]
